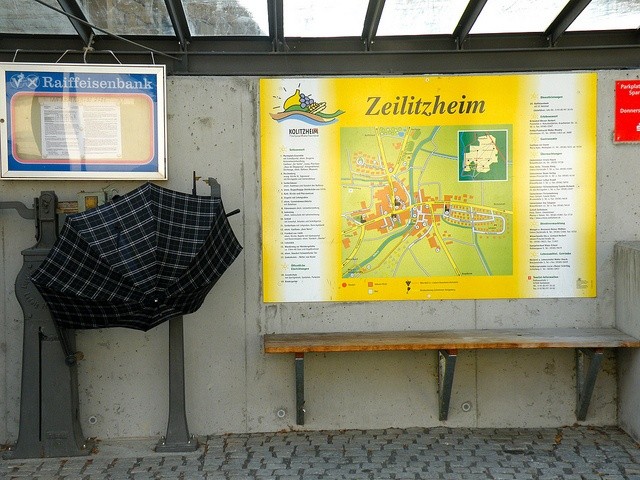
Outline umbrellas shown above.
[28,181,247,335]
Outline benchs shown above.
[264,326,640,426]
